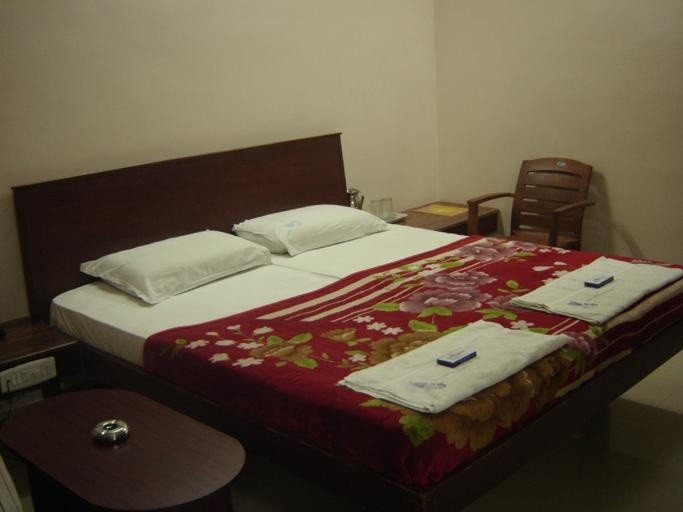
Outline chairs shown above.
[468,157,593,251]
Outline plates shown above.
[382,212,407,223]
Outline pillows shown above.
[229,204,391,255]
[79,229,272,306]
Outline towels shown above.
[511,255,681,326]
[337,320,571,416]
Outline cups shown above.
[369,200,382,217]
[381,197,394,221]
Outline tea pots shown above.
[346,189,365,210]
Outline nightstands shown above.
[390,199,498,235]
[0,314,79,405]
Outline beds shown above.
[10,133,682,512]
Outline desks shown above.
[0,389,245,510]
